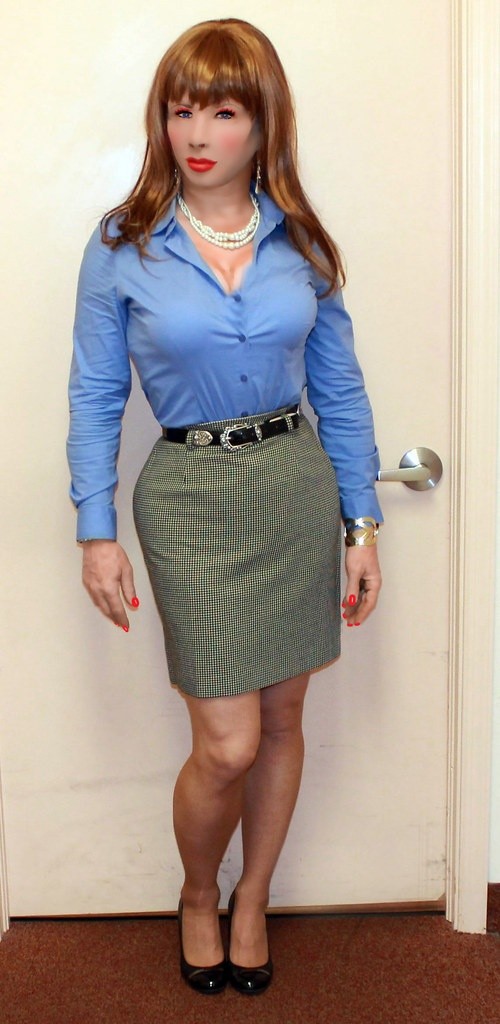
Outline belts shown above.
[162,406,300,452]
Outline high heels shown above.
[225,888,273,996]
[178,895,227,994]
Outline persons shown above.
[67,18,385,997]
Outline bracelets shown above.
[343,515,380,548]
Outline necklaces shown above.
[174,190,260,250]
